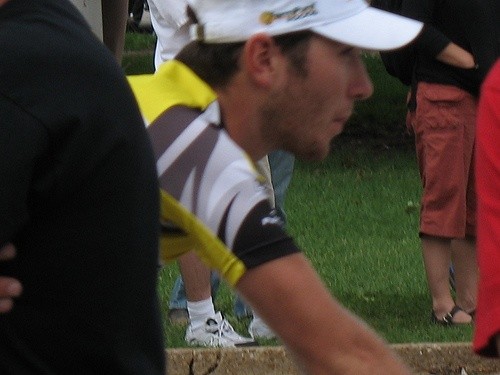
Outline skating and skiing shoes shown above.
[248,315,274,340]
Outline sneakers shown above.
[185,312,258,347]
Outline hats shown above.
[188,0,425,52]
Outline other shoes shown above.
[169,309,190,327]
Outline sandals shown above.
[431,305,474,325]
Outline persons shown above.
[406,0,500,324]
[0,0,426,375]
[473,60,500,361]
